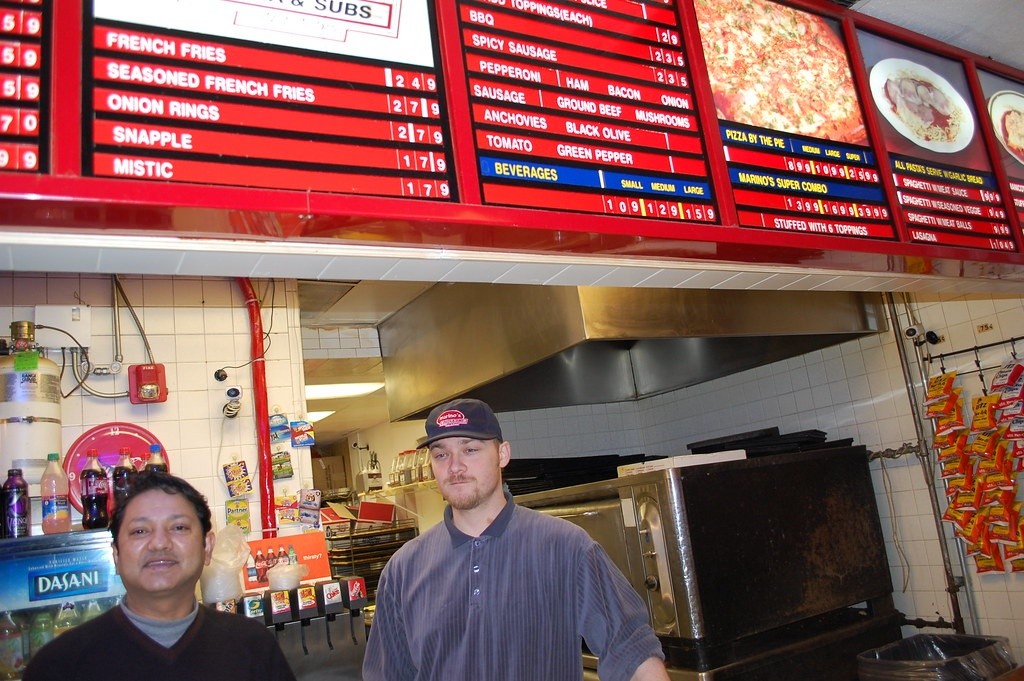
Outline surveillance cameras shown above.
[351,442,358,450]
[902,322,925,340]
[222,386,244,402]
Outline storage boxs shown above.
[320,508,350,542]
[327,501,397,540]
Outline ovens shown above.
[510,447,896,670]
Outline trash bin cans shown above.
[858,633,1018,680]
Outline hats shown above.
[416,398,504,449]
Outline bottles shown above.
[266,548,278,570]
[113,447,139,502]
[389,447,435,488]
[288,545,297,565]
[255,549,268,583]
[80,449,109,529]
[277,545,290,566]
[0,597,120,681]
[246,552,257,582]
[1,469,29,539]
[41,453,71,534]
[145,444,168,475]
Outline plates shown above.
[870,58,974,154]
[987,91,1024,166]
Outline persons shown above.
[21,474,296,681]
[361,399,671,681]
